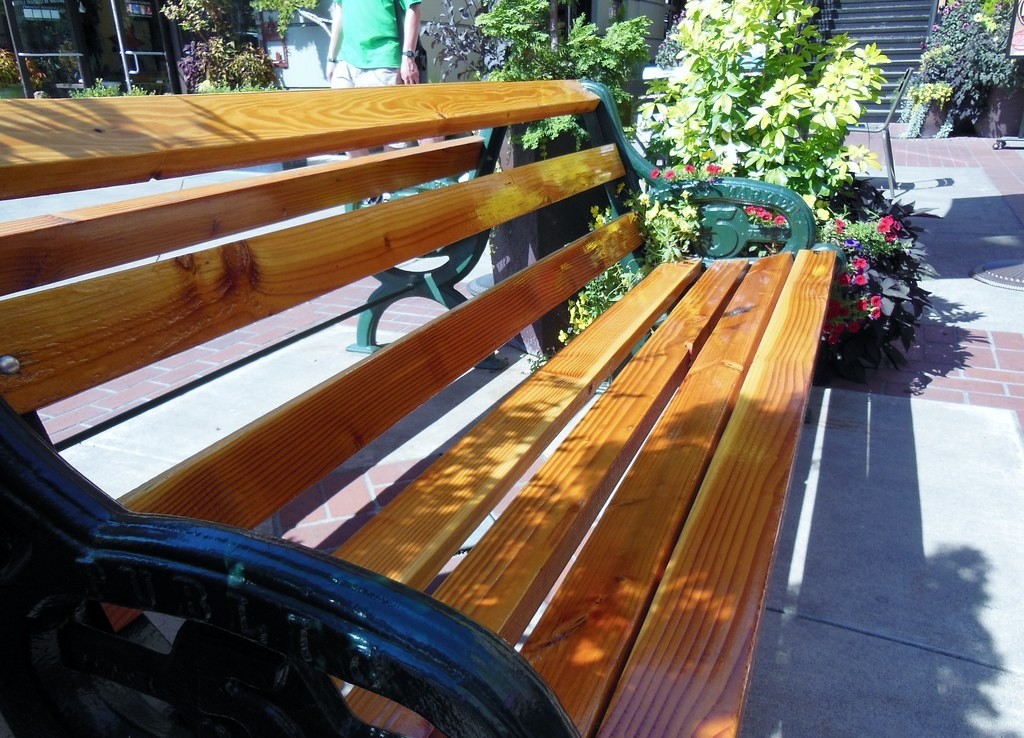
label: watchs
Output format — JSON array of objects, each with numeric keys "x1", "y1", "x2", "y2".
[{"x1": 402, "y1": 51, "x2": 416, "y2": 58}]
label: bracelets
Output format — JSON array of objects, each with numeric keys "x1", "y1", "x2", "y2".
[{"x1": 327, "y1": 58, "x2": 336, "y2": 62}]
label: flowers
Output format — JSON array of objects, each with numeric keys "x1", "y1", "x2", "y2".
[{"x1": 591, "y1": 163, "x2": 902, "y2": 347}]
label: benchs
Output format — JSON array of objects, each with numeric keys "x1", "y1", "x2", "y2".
[{"x1": 0, "y1": 81, "x2": 845, "y2": 738}]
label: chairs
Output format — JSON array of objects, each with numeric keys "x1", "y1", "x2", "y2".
[{"x1": 846, "y1": 68, "x2": 914, "y2": 198}]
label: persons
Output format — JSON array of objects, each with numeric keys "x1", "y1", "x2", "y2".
[{"x1": 326, "y1": 0, "x2": 423, "y2": 205}]
